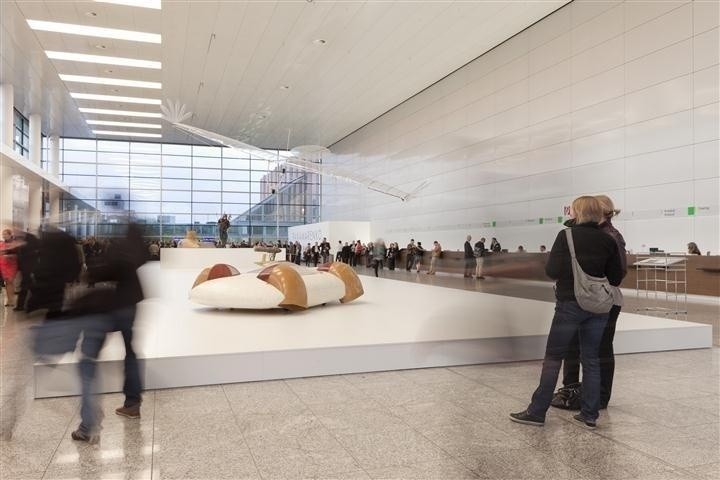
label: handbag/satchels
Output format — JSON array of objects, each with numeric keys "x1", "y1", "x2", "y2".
[
  {"x1": 551, "y1": 382, "x2": 582, "y2": 408},
  {"x1": 570, "y1": 261, "x2": 614, "y2": 314}
]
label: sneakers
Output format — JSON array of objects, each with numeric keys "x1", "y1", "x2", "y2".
[
  {"x1": 573, "y1": 414, "x2": 597, "y2": 430},
  {"x1": 509, "y1": 410, "x2": 545, "y2": 426},
  {"x1": 72, "y1": 431, "x2": 99, "y2": 443},
  {"x1": 116, "y1": 407, "x2": 140, "y2": 419}
]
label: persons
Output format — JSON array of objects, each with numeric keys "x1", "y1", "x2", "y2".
[
  {"x1": 688, "y1": 242, "x2": 701, "y2": 255},
  {"x1": 515, "y1": 245, "x2": 527, "y2": 253},
  {"x1": 335, "y1": 240, "x2": 362, "y2": 268},
  {"x1": 231, "y1": 240, "x2": 302, "y2": 264},
  {"x1": 509, "y1": 196, "x2": 621, "y2": 430},
  {"x1": 0, "y1": 215, "x2": 151, "y2": 445},
  {"x1": 149, "y1": 215, "x2": 230, "y2": 259},
  {"x1": 562, "y1": 196, "x2": 627, "y2": 409},
  {"x1": 304, "y1": 237, "x2": 330, "y2": 267},
  {"x1": 362, "y1": 239, "x2": 398, "y2": 277},
  {"x1": 463, "y1": 236, "x2": 500, "y2": 279},
  {"x1": 427, "y1": 241, "x2": 441, "y2": 275},
  {"x1": 406, "y1": 239, "x2": 424, "y2": 273},
  {"x1": 539, "y1": 245, "x2": 547, "y2": 253}
]
